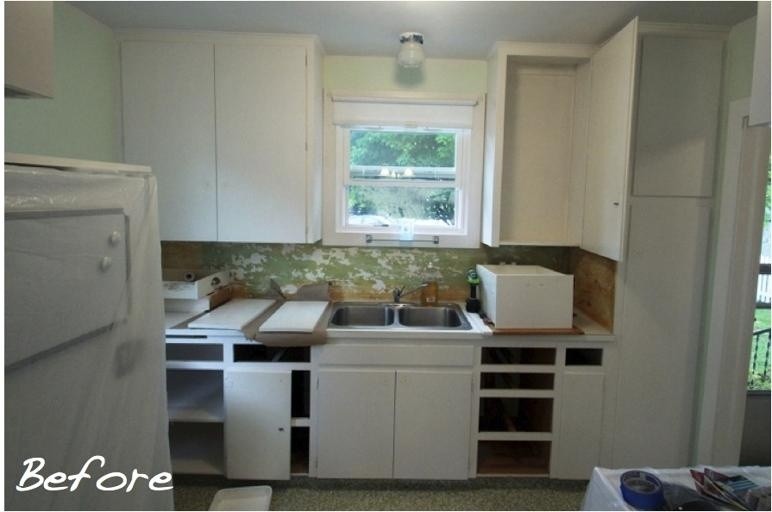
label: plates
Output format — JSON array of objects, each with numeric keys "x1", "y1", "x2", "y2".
[
  {"x1": 410, "y1": 167, "x2": 454, "y2": 178},
  {"x1": 350, "y1": 164, "x2": 381, "y2": 177}
]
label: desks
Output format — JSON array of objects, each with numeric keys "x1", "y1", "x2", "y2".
[{"x1": 579, "y1": 465, "x2": 770, "y2": 511}]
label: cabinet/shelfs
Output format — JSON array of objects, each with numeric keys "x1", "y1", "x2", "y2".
[
  {"x1": 314, "y1": 365, "x2": 475, "y2": 482},
  {"x1": 482, "y1": 14, "x2": 639, "y2": 264},
  {"x1": 164, "y1": 334, "x2": 313, "y2": 481},
  {"x1": 113, "y1": 27, "x2": 323, "y2": 245},
  {"x1": 478, "y1": 344, "x2": 612, "y2": 482},
  {"x1": 572, "y1": 32, "x2": 727, "y2": 470}
]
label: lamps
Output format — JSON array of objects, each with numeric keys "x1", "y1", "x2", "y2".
[{"x1": 397, "y1": 31, "x2": 426, "y2": 70}]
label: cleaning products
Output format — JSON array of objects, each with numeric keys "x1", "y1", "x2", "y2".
[{"x1": 465, "y1": 269, "x2": 481, "y2": 312}]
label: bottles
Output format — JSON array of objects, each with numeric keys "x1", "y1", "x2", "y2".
[{"x1": 420, "y1": 275, "x2": 438, "y2": 306}]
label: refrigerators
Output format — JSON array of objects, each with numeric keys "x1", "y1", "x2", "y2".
[{"x1": 5, "y1": 151, "x2": 176, "y2": 511}]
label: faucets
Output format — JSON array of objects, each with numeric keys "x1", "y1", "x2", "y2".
[{"x1": 392, "y1": 283, "x2": 430, "y2": 303}]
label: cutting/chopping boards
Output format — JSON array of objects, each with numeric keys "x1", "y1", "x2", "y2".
[
  {"x1": 257, "y1": 300, "x2": 329, "y2": 333},
  {"x1": 185, "y1": 298, "x2": 277, "y2": 331}
]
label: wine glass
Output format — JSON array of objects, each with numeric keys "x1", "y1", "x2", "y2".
[{"x1": 386, "y1": 166, "x2": 405, "y2": 179}]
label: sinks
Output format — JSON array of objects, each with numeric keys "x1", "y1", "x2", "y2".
[
  {"x1": 328, "y1": 301, "x2": 395, "y2": 329},
  {"x1": 398, "y1": 302, "x2": 471, "y2": 330}
]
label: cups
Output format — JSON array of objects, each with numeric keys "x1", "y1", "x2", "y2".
[{"x1": 398, "y1": 218, "x2": 415, "y2": 241}]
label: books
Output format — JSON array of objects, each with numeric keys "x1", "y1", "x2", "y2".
[{"x1": 690, "y1": 466, "x2": 771, "y2": 511}]
